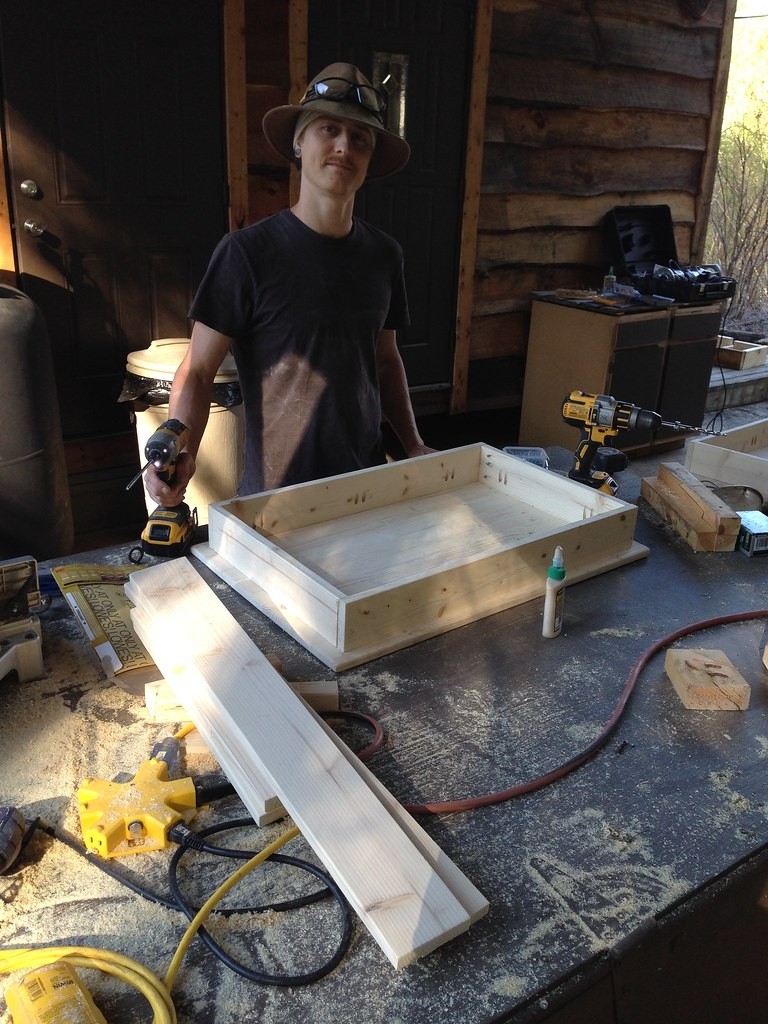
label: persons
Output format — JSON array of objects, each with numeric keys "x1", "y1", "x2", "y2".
[{"x1": 142, "y1": 62, "x2": 439, "y2": 508}]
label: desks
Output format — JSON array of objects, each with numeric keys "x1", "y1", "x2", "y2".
[{"x1": 0, "y1": 445, "x2": 768, "y2": 1024}]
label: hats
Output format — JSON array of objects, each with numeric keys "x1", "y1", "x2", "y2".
[{"x1": 262, "y1": 62, "x2": 411, "y2": 186}]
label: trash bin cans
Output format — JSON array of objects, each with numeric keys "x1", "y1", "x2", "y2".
[{"x1": 124, "y1": 335, "x2": 246, "y2": 529}]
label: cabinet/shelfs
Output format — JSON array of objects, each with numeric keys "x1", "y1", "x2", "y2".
[{"x1": 518, "y1": 291, "x2": 726, "y2": 459}]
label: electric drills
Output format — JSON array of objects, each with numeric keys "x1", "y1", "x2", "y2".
[
  {"x1": 126, "y1": 419, "x2": 199, "y2": 565},
  {"x1": 560, "y1": 389, "x2": 727, "y2": 496}
]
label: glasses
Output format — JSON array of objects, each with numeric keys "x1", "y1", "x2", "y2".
[{"x1": 306, "y1": 77, "x2": 387, "y2": 114}]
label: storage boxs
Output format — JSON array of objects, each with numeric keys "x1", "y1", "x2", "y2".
[
  {"x1": 684, "y1": 418, "x2": 768, "y2": 496},
  {"x1": 713, "y1": 335, "x2": 768, "y2": 370},
  {"x1": 604, "y1": 204, "x2": 738, "y2": 303},
  {"x1": 736, "y1": 511, "x2": 768, "y2": 558},
  {"x1": 190, "y1": 441, "x2": 651, "y2": 673}
]
